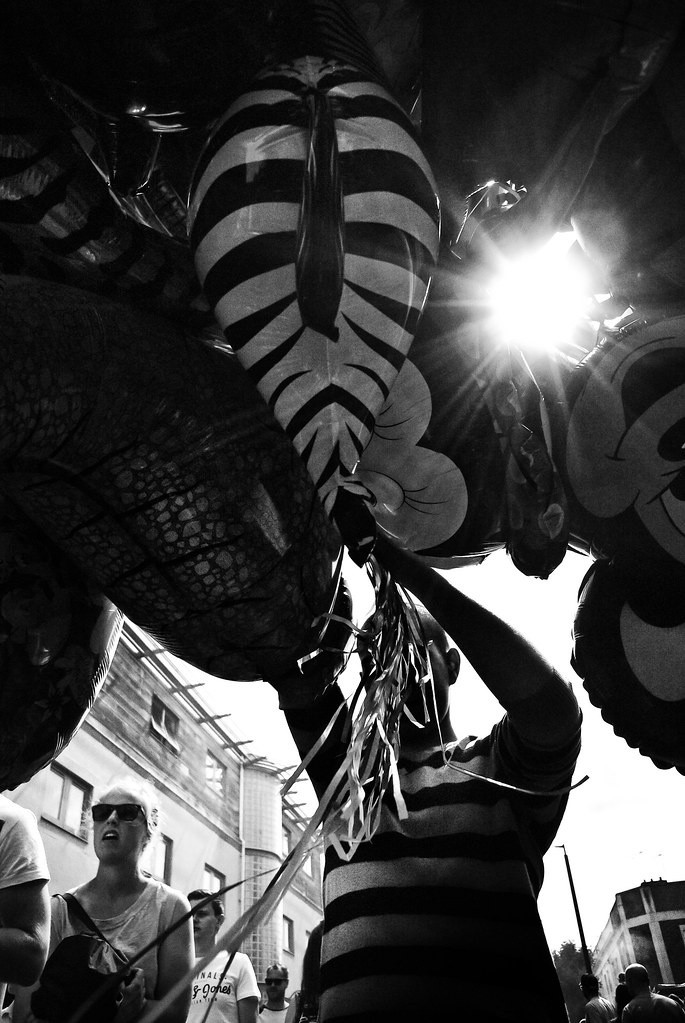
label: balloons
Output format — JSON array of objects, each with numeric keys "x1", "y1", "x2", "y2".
[{"x1": 0, "y1": 1, "x2": 684, "y2": 798}]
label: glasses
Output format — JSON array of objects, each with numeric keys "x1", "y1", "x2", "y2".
[
  {"x1": 91, "y1": 804, "x2": 145, "y2": 822},
  {"x1": 265, "y1": 978, "x2": 288, "y2": 986}
]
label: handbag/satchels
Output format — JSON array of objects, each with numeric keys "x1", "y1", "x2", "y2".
[{"x1": 30, "y1": 934, "x2": 135, "y2": 1023}]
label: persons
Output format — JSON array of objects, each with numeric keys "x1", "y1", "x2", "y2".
[
  {"x1": 261, "y1": 500, "x2": 582, "y2": 1022},
  {"x1": 567, "y1": 962, "x2": 685, "y2": 1023},
  {"x1": 185, "y1": 886, "x2": 261, "y2": 1023},
  {"x1": 258, "y1": 963, "x2": 291, "y2": 1023},
  {"x1": 0, "y1": 798, "x2": 51, "y2": 1023},
  {"x1": 51, "y1": 788, "x2": 197, "y2": 1023}
]
